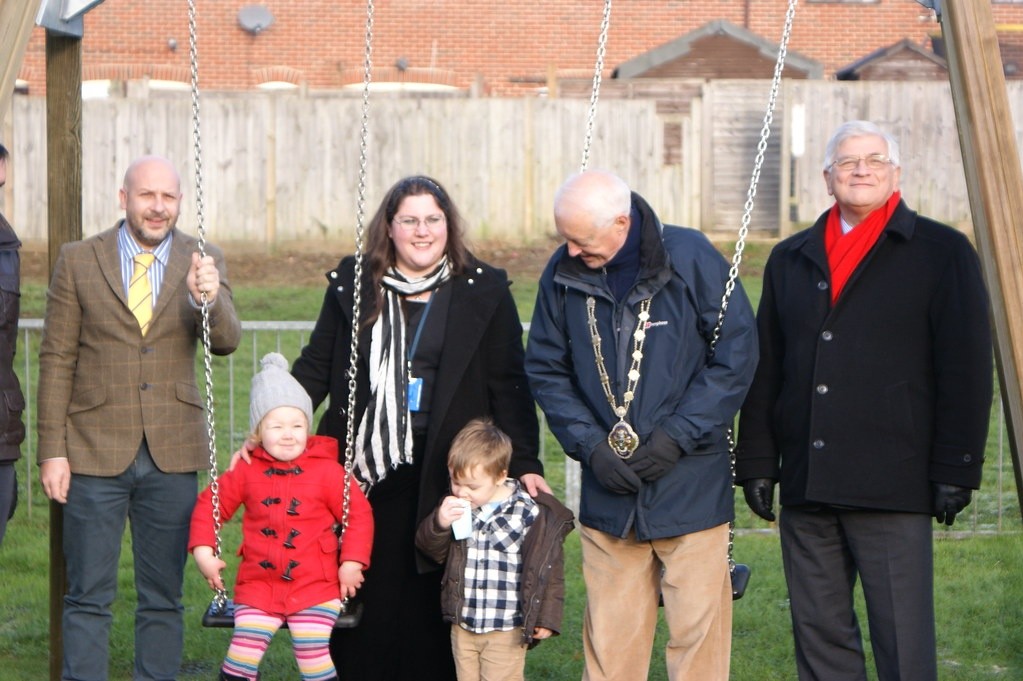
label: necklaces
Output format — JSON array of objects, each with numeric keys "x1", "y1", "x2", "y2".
[
  {"x1": 415, "y1": 293, "x2": 422, "y2": 300},
  {"x1": 585, "y1": 292, "x2": 653, "y2": 459}
]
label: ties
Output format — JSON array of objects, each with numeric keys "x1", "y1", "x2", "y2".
[{"x1": 128, "y1": 253, "x2": 155, "y2": 337}]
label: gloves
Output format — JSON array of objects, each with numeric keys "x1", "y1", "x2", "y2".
[
  {"x1": 742, "y1": 478, "x2": 775, "y2": 521},
  {"x1": 932, "y1": 482, "x2": 972, "y2": 525},
  {"x1": 589, "y1": 441, "x2": 643, "y2": 494},
  {"x1": 625, "y1": 431, "x2": 682, "y2": 483}
]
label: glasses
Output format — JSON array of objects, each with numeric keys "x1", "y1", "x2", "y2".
[
  {"x1": 828, "y1": 153, "x2": 896, "y2": 170},
  {"x1": 392, "y1": 214, "x2": 447, "y2": 231}
]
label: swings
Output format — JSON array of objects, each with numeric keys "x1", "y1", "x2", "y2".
[
  {"x1": 579, "y1": 0, "x2": 798, "y2": 607},
  {"x1": 188, "y1": 0, "x2": 381, "y2": 629}
]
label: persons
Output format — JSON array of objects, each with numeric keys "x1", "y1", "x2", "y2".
[
  {"x1": 413, "y1": 419, "x2": 575, "y2": 681},
  {"x1": 734, "y1": 121, "x2": 993, "y2": 681},
  {"x1": 225, "y1": 176, "x2": 555, "y2": 681},
  {"x1": 36, "y1": 155, "x2": 241, "y2": 681},
  {"x1": 523, "y1": 170, "x2": 760, "y2": 681},
  {"x1": 0, "y1": 144, "x2": 25, "y2": 542},
  {"x1": 188, "y1": 351, "x2": 373, "y2": 681}
]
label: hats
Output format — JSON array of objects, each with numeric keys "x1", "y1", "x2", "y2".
[{"x1": 249, "y1": 352, "x2": 312, "y2": 435}]
